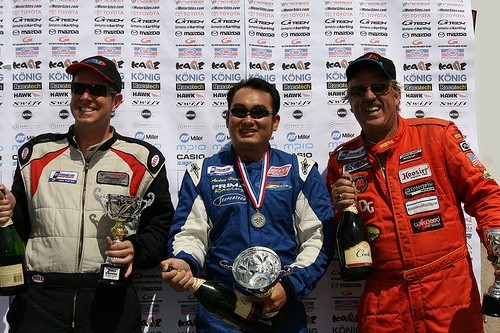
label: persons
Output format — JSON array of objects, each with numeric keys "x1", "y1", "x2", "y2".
[
  {"x1": 160, "y1": 77, "x2": 335, "y2": 333},
  {"x1": 0, "y1": 55, "x2": 175, "y2": 333},
  {"x1": 326, "y1": 52, "x2": 500, "y2": 333}
]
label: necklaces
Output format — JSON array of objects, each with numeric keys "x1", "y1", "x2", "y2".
[{"x1": 365, "y1": 138, "x2": 376, "y2": 144}]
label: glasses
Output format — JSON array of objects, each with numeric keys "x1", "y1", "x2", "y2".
[
  {"x1": 70, "y1": 82, "x2": 116, "y2": 97},
  {"x1": 229, "y1": 108, "x2": 273, "y2": 119},
  {"x1": 349, "y1": 84, "x2": 391, "y2": 98}
]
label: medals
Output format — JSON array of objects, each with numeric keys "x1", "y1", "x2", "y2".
[{"x1": 251, "y1": 213, "x2": 266, "y2": 228}]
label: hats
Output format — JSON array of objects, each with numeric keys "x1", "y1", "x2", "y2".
[
  {"x1": 66, "y1": 56, "x2": 122, "y2": 93},
  {"x1": 346, "y1": 52, "x2": 396, "y2": 84}
]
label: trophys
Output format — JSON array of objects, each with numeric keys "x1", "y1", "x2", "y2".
[
  {"x1": 220, "y1": 245, "x2": 293, "y2": 318},
  {"x1": 93, "y1": 187, "x2": 155, "y2": 284}
]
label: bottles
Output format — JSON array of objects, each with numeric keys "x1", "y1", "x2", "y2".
[
  {"x1": 337, "y1": 177, "x2": 373, "y2": 281},
  {"x1": 0, "y1": 190, "x2": 29, "y2": 296},
  {"x1": 166, "y1": 265, "x2": 263, "y2": 330}
]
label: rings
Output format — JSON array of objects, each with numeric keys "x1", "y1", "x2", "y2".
[{"x1": 336, "y1": 193, "x2": 342, "y2": 201}]
